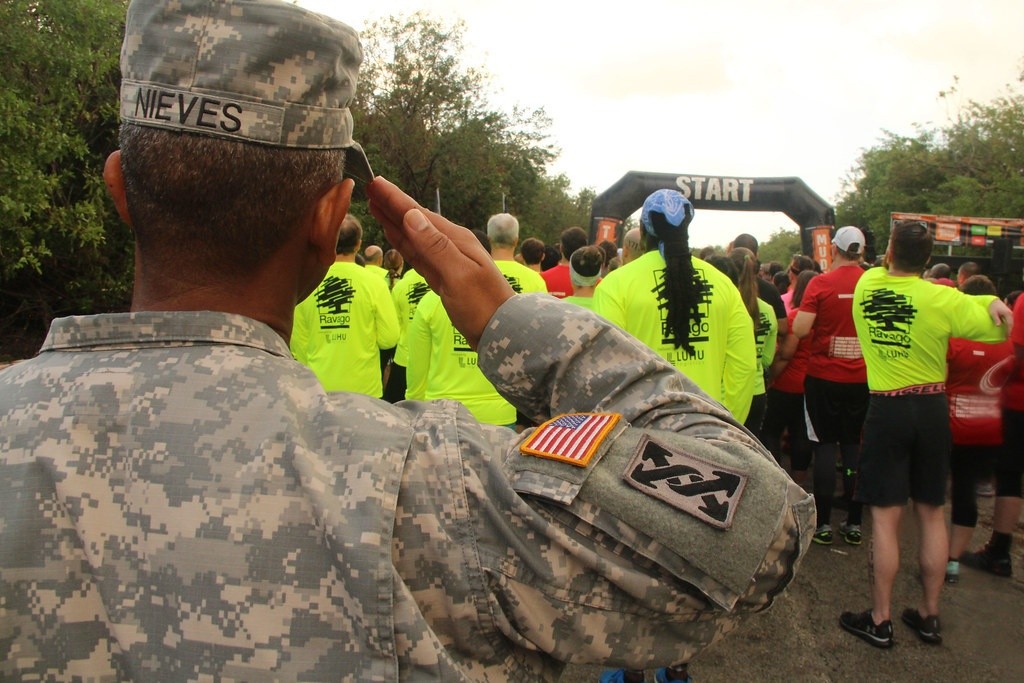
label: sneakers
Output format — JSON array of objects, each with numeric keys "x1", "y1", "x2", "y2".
[
  {"x1": 599, "y1": 668, "x2": 644, "y2": 683},
  {"x1": 901, "y1": 606, "x2": 941, "y2": 643},
  {"x1": 841, "y1": 610, "x2": 893, "y2": 648},
  {"x1": 654, "y1": 665, "x2": 690, "y2": 683}
]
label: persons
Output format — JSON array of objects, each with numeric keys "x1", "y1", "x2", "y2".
[
  {"x1": 593, "y1": 188, "x2": 757, "y2": 683},
  {"x1": 701, "y1": 234, "x2": 824, "y2": 487},
  {"x1": 516, "y1": 227, "x2": 642, "y2": 308},
  {"x1": 862, "y1": 229, "x2": 885, "y2": 267},
  {"x1": 405, "y1": 230, "x2": 517, "y2": 432},
  {"x1": 289, "y1": 213, "x2": 400, "y2": 399},
  {"x1": 792, "y1": 225, "x2": 866, "y2": 544},
  {"x1": 959, "y1": 290, "x2": 1024, "y2": 577},
  {"x1": 839, "y1": 219, "x2": 1014, "y2": 648},
  {"x1": 355, "y1": 245, "x2": 431, "y2": 403},
  {"x1": 923, "y1": 262, "x2": 981, "y2": 286},
  {"x1": 913, "y1": 276, "x2": 1017, "y2": 584},
  {"x1": 0, "y1": 0, "x2": 816, "y2": 683},
  {"x1": 486, "y1": 213, "x2": 547, "y2": 297}
]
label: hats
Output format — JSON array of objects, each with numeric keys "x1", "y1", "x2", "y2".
[
  {"x1": 118, "y1": 1, "x2": 376, "y2": 184},
  {"x1": 829, "y1": 228, "x2": 865, "y2": 256}
]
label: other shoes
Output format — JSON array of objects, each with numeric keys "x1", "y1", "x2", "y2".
[
  {"x1": 979, "y1": 543, "x2": 1013, "y2": 576},
  {"x1": 946, "y1": 561, "x2": 960, "y2": 584},
  {"x1": 812, "y1": 528, "x2": 832, "y2": 544},
  {"x1": 837, "y1": 523, "x2": 862, "y2": 545}
]
